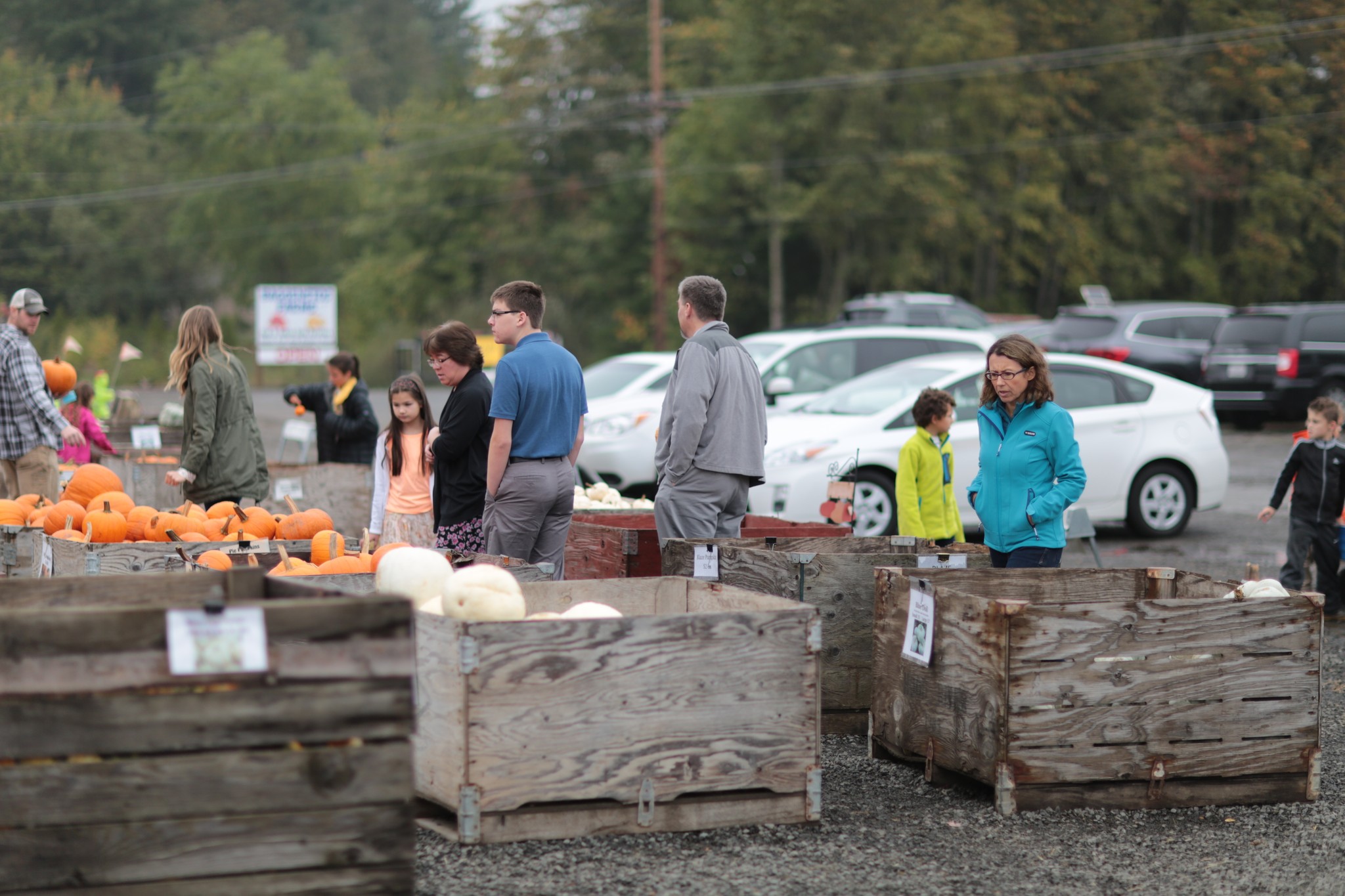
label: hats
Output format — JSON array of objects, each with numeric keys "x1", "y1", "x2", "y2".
[{"x1": 9, "y1": 288, "x2": 50, "y2": 316}]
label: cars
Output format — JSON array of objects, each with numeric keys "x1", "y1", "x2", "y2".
[{"x1": 746, "y1": 351, "x2": 1233, "y2": 552}]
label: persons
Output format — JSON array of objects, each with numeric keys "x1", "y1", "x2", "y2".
[
  {"x1": 653, "y1": 273, "x2": 769, "y2": 554},
  {"x1": 481, "y1": 281, "x2": 587, "y2": 581},
  {"x1": 0, "y1": 287, "x2": 86, "y2": 504},
  {"x1": 282, "y1": 353, "x2": 378, "y2": 469},
  {"x1": 423, "y1": 319, "x2": 494, "y2": 555},
  {"x1": 967, "y1": 334, "x2": 1087, "y2": 569},
  {"x1": 360, "y1": 373, "x2": 438, "y2": 551},
  {"x1": 59, "y1": 384, "x2": 116, "y2": 466},
  {"x1": 1257, "y1": 397, "x2": 1345, "y2": 621},
  {"x1": 164, "y1": 303, "x2": 271, "y2": 516},
  {"x1": 1292, "y1": 387, "x2": 1345, "y2": 593},
  {"x1": 895, "y1": 387, "x2": 966, "y2": 548}
]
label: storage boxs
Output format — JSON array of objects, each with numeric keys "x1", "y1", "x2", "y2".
[
  {"x1": 407, "y1": 501, "x2": 1328, "y2": 842},
  {"x1": 1, "y1": 534, "x2": 418, "y2": 895}
]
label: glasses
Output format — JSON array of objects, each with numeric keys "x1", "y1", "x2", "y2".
[
  {"x1": 427, "y1": 356, "x2": 453, "y2": 367},
  {"x1": 984, "y1": 368, "x2": 1028, "y2": 380},
  {"x1": 491, "y1": 311, "x2": 528, "y2": 317}
]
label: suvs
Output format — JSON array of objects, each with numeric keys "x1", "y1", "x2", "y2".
[
  {"x1": 1197, "y1": 303, "x2": 1345, "y2": 425},
  {"x1": 573, "y1": 326, "x2": 1002, "y2": 499},
  {"x1": 1045, "y1": 303, "x2": 1236, "y2": 387},
  {"x1": 843, "y1": 293, "x2": 992, "y2": 334}
]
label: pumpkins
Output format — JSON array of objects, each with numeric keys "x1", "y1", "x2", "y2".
[
  {"x1": 38, "y1": 356, "x2": 77, "y2": 401},
  {"x1": 1, "y1": 470, "x2": 410, "y2": 666}
]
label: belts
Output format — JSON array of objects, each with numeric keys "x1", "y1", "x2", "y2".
[{"x1": 510, "y1": 456, "x2": 558, "y2": 464}]
